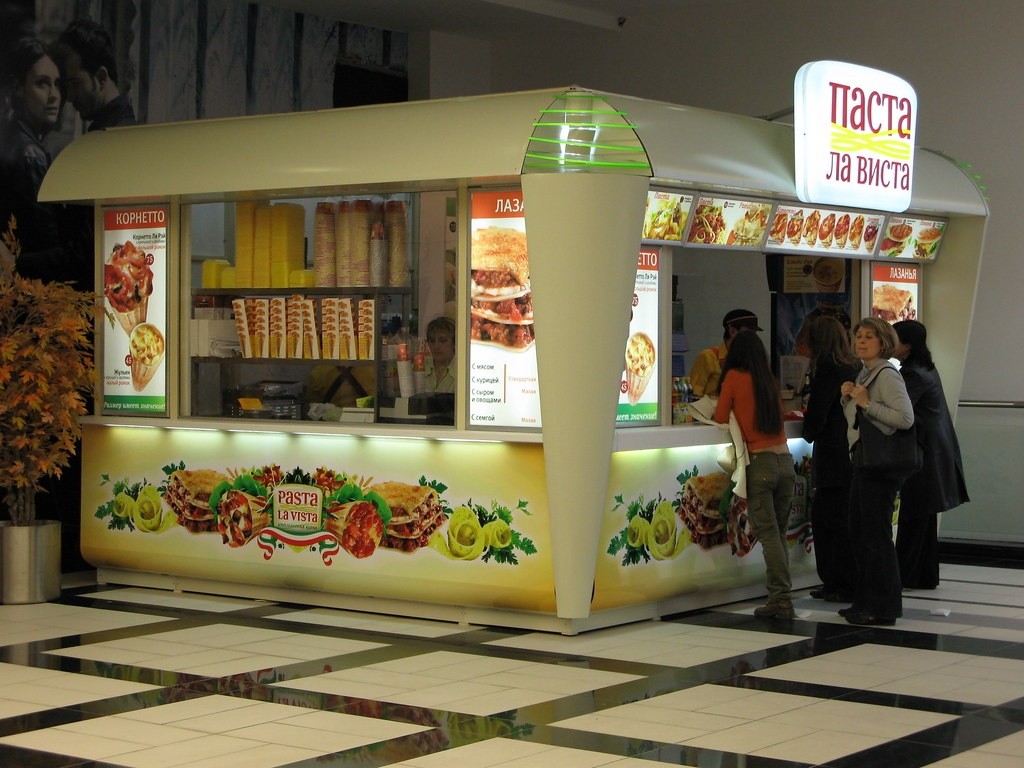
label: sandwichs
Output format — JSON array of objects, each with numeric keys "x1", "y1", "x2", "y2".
[
  {"x1": 871, "y1": 285, "x2": 917, "y2": 325},
  {"x1": 165, "y1": 470, "x2": 237, "y2": 535},
  {"x1": 678, "y1": 472, "x2": 731, "y2": 550},
  {"x1": 360, "y1": 480, "x2": 447, "y2": 553}
]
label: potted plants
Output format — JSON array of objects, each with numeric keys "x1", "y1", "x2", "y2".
[{"x1": 0, "y1": 214, "x2": 120, "y2": 606}]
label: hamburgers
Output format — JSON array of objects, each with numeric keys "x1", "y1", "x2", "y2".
[
  {"x1": 880, "y1": 224, "x2": 942, "y2": 259},
  {"x1": 467, "y1": 225, "x2": 535, "y2": 353}
]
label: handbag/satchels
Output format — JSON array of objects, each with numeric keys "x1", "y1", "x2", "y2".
[{"x1": 853, "y1": 366, "x2": 918, "y2": 470}]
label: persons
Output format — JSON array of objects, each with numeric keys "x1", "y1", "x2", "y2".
[
  {"x1": 715, "y1": 331, "x2": 796, "y2": 621},
  {"x1": 689, "y1": 310, "x2": 764, "y2": 396},
  {"x1": 838, "y1": 317, "x2": 915, "y2": 625},
  {"x1": 891, "y1": 320, "x2": 970, "y2": 590},
  {"x1": 427, "y1": 316, "x2": 456, "y2": 391},
  {"x1": 0, "y1": 17, "x2": 135, "y2": 248},
  {"x1": 306, "y1": 364, "x2": 377, "y2": 406},
  {"x1": 802, "y1": 315, "x2": 862, "y2": 601}
]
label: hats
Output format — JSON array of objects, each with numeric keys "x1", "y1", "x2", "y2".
[{"x1": 724, "y1": 309, "x2": 764, "y2": 331}]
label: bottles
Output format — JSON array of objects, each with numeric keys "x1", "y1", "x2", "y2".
[{"x1": 672, "y1": 376, "x2": 694, "y2": 425}]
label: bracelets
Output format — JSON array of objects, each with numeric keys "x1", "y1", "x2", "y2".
[{"x1": 863, "y1": 402, "x2": 870, "y2": 409}]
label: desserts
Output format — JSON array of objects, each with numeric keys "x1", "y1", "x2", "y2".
[
  {"x1": 623, "y1": 331, "x2": 656, "y2": 407},
  {"x1": 769, "y1": 210, "x2": 880, "y2": 254},
  {"x1": 212, "y1": 465, "x2": 384, "y2": 558},
  {"x1": 104, "y1": 240, "x2": 154, "y2": 338}
]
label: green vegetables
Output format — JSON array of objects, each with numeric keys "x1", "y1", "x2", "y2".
[
  {"x1": 93, "y1": 459, "x2": 188, "y2": 532},
  {"x1": 417, "y1": 477, "x2": 538, "y2": 566},
  {"x1": 604, "y1": 465, "x2": 700, "y2": 566}
]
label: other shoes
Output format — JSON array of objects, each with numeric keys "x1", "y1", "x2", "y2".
[
  {"x1": 810, "y1": 587, "x2": 857, "y2": 603},
  {"x1": 754, "y1": 594, "x2": 794, "y2": 620},
  {"x1": 838, "y1": 604, "x2": 865, "y2": 616},
  {"x1": 846, "y1": 609, "x2": 896, "y2": 626}
]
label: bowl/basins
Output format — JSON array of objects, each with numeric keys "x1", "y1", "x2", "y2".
[
  {"x1": 314, "y1": 199, "x2": 409, "y2": 288},
  {"x1": 812, "y1": 257, "x2": 844, "y2": 293}
]
